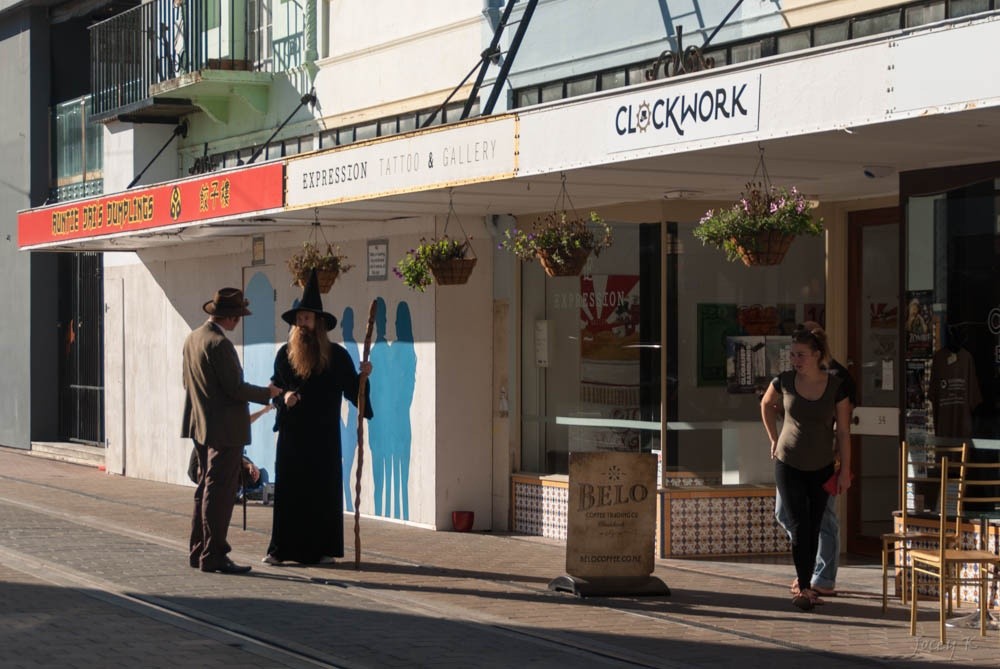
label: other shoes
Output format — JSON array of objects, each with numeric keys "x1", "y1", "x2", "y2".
[
  {"x1": 262, "y1": 553, "x2": 285, "y2": 565},
  {"x1": 319, "y1": 556, "x2": 336, "y2": 563}
]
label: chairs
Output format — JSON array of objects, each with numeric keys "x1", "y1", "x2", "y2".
[
  {"x1": 877, "y1": 438, "x2": 969, "y2": 619},
  {"x1": 907, "y1": 456, "x2": 1000, "y2": 646}
]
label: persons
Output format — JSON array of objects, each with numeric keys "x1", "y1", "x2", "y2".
[
  {"x1": 179, "y1": 287, "x2": 283, "y2": 574},
  {"x1": 187, "y1": 443, "x2": 260, "y2": 501},
  {"x1": 799, "y1": 319, "x2": 849, "y2": 597},
  {"x1": 760, "y1": 326, "x2": 848, "y2": 610},
  {"x1": 261, "y1": 274, "x2": 373, "y2": 566}
]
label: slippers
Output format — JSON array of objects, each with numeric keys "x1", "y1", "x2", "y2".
[
  {"x1": 791, "y1": 584, "x2": 800, "y2": 593},
  {"x1": 806, "y1": 591, "x2": 825, "y2": 604},
  {"x1": 792, "y1": 592, "x2": 815, "y2": 611},
  {"x1": 811, "y1": 587, "x2": 837, "y2": 597}
]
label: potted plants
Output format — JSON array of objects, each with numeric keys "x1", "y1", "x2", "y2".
[
  {"x1": 495, "y1": 206, "x2": 614, "y2": 278},
  {"x1": 396, "y1": 235, "x2": 479, "y2": 292},
  {"x1": 283, "y1": 239, "x2": 354, "y2": 295},
  {"x1": 693, "y1": 183, "x2": 826, "y2": 267}
]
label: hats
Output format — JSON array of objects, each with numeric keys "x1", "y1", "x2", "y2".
[
  {"x1": 280, "y1": 270, "x2": 338, "y2": 333},
  {"x1": 202, "y1": 288, "x2": 254, "y2": 318}
]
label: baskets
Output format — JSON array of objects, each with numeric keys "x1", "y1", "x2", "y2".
[
  {"x1": 427, "y1": 255, "x2": 478, "y2": 286},
  {"x1": 292, "y1": 267, "x2": 342, "y2": 294},
  {"x1": 726, "y1": 230, "x2": 798, "y2": 266},
  {"x1": 533, "y1": 237, "x2": 590, "y2": 277}
]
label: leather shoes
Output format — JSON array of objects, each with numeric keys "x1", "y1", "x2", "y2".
[{"x1": 201, "y1": 557, "x2": 252, "y2": 574}]
